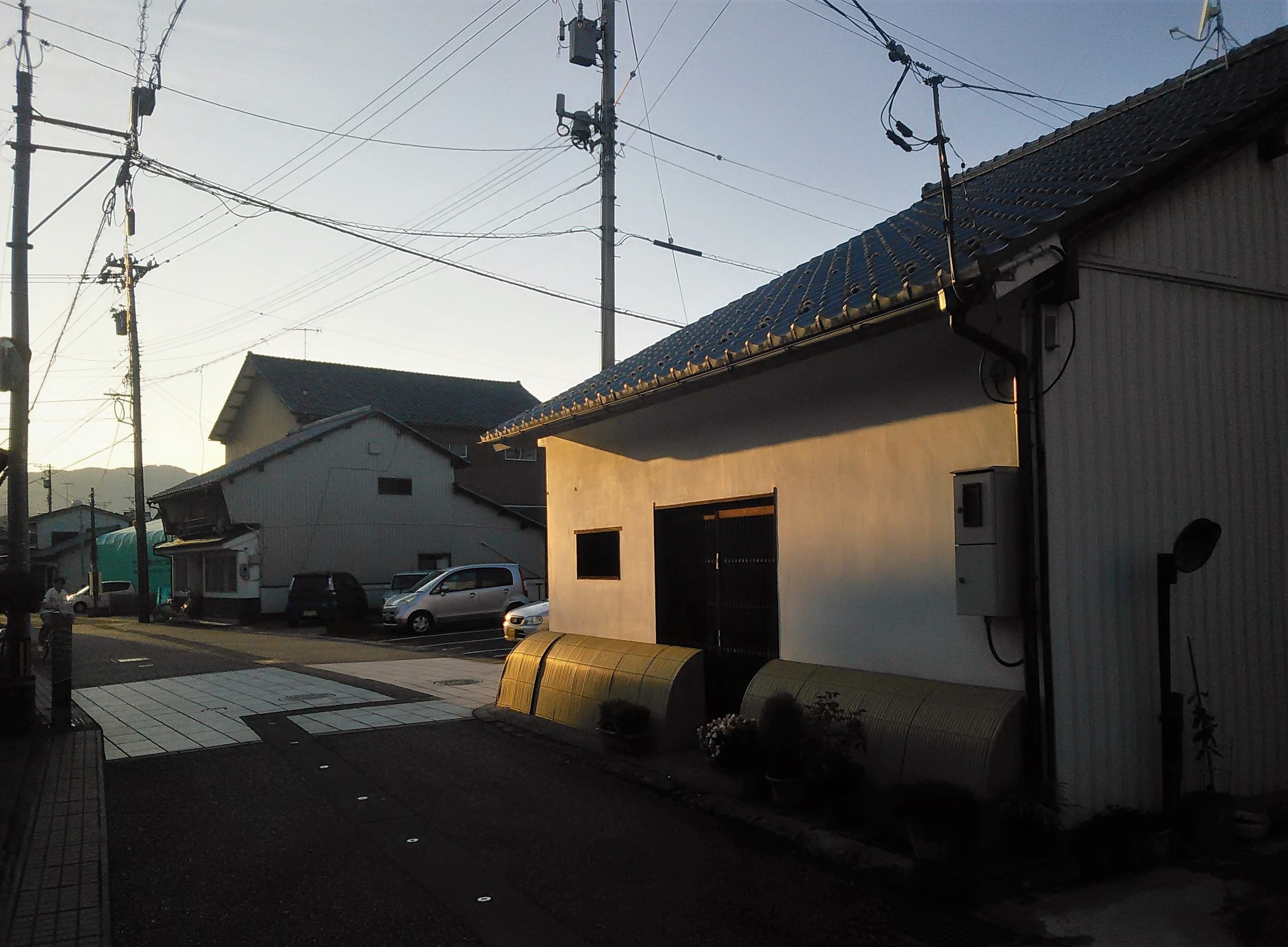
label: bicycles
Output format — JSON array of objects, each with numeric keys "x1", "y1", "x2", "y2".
[{"x1": 39, "y1": 608, "x2": 61, "y2": 659}]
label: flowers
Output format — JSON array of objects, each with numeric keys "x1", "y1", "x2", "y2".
[{"x1": 696, "y1": 713, "x2": 760, "y2": 774}]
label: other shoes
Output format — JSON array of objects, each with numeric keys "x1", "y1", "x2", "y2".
[{"x1": 38, "y1": 645, "x2": 43, "y2": 652}]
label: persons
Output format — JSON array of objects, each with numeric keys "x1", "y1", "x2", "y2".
[{"x1": 37, "y1": 577, "x2": 68, "y2": 653}]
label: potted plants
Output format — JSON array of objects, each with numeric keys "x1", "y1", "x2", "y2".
[
  {"x1": 596, "y1": 697, "x2": 652, "y2": 756},
  {"x1": 1179, "y1": 634, "x2": 1237, "y2": 852},
  {"x1": 757, "y1": 688, "x2": 982, "y2": 866},
  {"x1": 1234, "y1": 809, "x2": 1268, "y2": 842},
  {"x1": 1092, "y1": 803, "x2": 1171, "y2": 868}
]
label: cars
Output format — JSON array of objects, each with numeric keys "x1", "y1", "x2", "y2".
[
  {"x1": 284, "y1": 570, "x2": 369, "y2": 629},
  {"x1": 71, "y1": 580, "x2": 136, "y2": 614},
  {"x1": 379, "y1": 562, "x2": 531, "y2": 637},
  {"x1": 502, "y1": 597, "x2": 549, "y2": 643}
]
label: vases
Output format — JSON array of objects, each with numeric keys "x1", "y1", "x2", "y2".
[{"x1": 730, "y1": 772, "x2": 767, "y2": 806}]
